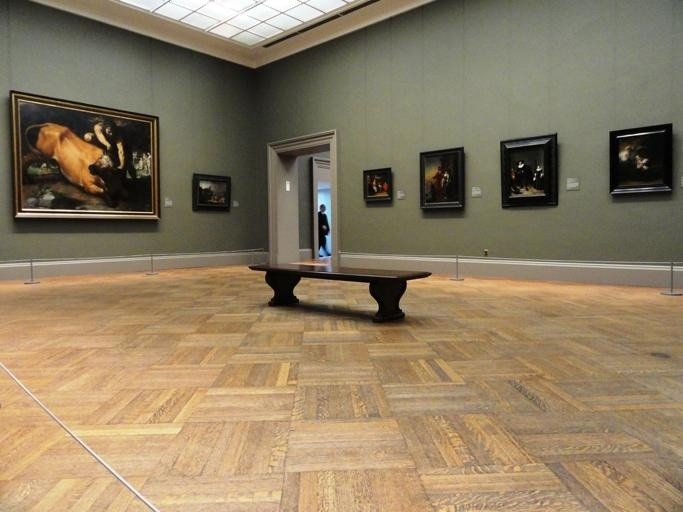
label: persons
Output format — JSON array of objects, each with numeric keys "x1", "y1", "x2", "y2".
[
  {"x1": 83, "y1": 119, "x2": 125, "y2": 169},
  {"x1": 370, "y1": 145, "x2": 652, "y2": 199},
  {"x1": 318, "y1": 204, "x2": 331, "y2": 256}
]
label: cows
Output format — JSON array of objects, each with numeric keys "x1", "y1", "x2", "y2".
[{"x1": 22, "y1": 122, "x2": 117, "y2": 208}]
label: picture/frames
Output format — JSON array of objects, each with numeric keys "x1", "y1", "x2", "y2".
[
  {"x1": 419, "y1": 146, "x2": 466, "y2": 211},
  {"x1": 362, "y1": 167, "x2": 393, "y2": 203},
  {"x1": 499, "y1": 132, "x2": 559, "y2": 209},
  {"x1": 8, "y1": 89, "x2": 162, "y2": 221},
  {"x1": 608, "y1": 121, "x2": 675, "y2": 198},
  {"x1": 192, "y1": 172, "x2": 231, "y2": 211}
]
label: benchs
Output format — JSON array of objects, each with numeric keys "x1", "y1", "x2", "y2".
[{"x1": 246, "y1": 262, "x2": 432, "y2": 324}]
